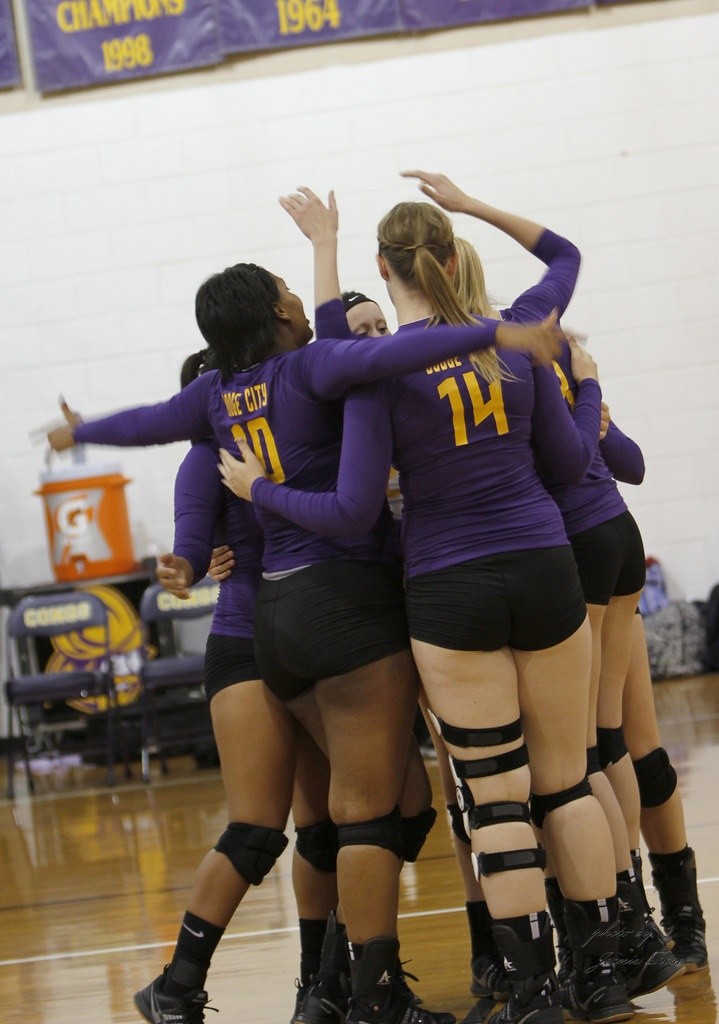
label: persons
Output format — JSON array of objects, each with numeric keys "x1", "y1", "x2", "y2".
[
  {"x1": 51, "y1": 265, "x2": 568, "y2": 1024},
  {"x1": 133, "y1": 172, "x2": 708, "y2": 1024}
]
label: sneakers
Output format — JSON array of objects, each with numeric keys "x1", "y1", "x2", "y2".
[{"x1": 133, "y1": 850, "x2": 710, "y2": 1024}]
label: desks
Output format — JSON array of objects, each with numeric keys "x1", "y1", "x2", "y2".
[{"x1": 0, "y1": 566, "x2": 179, "y2": 754}]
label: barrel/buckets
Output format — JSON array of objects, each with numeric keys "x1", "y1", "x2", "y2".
[{"x1": 32, "y1": 442, "x2": 137, "y2": 581}]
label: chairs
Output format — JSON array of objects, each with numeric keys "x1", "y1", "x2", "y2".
[
  {"x1": 4, "y1": 593, "x2": 119, "y2": 801},
  {"x1": 137, "y1": 574, "x2": 222, "y2": 780}
]
